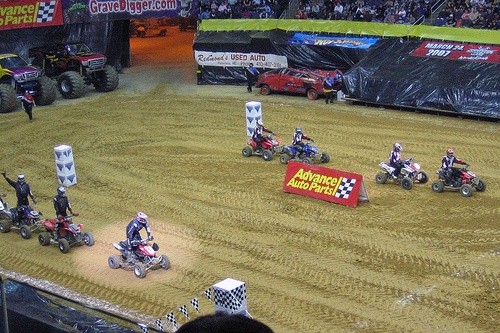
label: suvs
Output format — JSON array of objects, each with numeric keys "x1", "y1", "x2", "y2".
[
  {"x1": 0, "y1": 53, "x2": 56, "y2": 113},
  {"x1": 29, "y1": 42, "x2": 119, "y2": 99}
]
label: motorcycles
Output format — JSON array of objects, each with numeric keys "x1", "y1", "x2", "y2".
[
  {"x1": 1, "y1": 206, "x2": 45, "y2": 240},
  {"x1": 280, "y1": 139, "x2": 329, "y2": 166},
  {"x1": 108, "y1": 237, "x2": 171, "y2": 277},
  {"x1": 242, "y1": 132, "x2": 283, "y2": 160},
  {"x1": 431, "y1": 164, "x2": 487, "y2": 197},
  {"x1": 38, "y1": 213, "x2": 95, "y2": 255},
  {"x1": 375, "y1": 159, "x2": 429, "y2": 190}
]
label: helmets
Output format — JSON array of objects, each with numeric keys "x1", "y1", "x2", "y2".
[
  {"x1": 295, "y1": 128, "x2": 303, "y2": 133},
  {"x1": 136, "y1": 212, "x2": 148, "y2": 224},
  {"x1": 256, "y1": 120, "x2": 264, "y2": 127},
  {"x1": 17, "y1": 174, "x2": 25, "y2": 182},
  {"x1": 57, "y1": 186, "x2": 65, "y2": 198},
  {"x1": 446, "y1": 148, "x2": 454, "y2": 154},
  {"x1": 394, "y1": 143, "x2": 401, "y2": 150}
]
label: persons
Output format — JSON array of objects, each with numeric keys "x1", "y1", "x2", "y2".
[
  {"x1": 1, "y1": 171, "x2": 39, "y2": 209},
  {"x1": 21, "y1": 90, "x2": 36, "y2": 120},
  {"x1": 126, "y1": 212, "x2": 154, "y2": 250},
  {"x1": 176, "y1": 0, "x2": 500, "y2": 36},
  {"x1": 323, "y1": 72, "x2": 334, "y2": 103},
  {"x1": 442, "y1": 147, "x2": 470, "y2": 185},
  {"x1": 253, "y1": 119, "x2": 274, "y2": 149},
  {"x1": 246, "y1": 62, "x2": 260, "y2": 93},
  {"x1": 292, "y1": 126, "x2": 314, "y2": 154},
  {"x1": 53, "y1": 186, "x2": 79, "y2": 219},
  {"x1": 390, "y1": 143, "x2": 409, "y2": 179}
]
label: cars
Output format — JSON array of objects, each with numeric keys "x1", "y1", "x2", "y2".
[{"x1": 256, "y1": 68, "x2": 324, "y2": 100}]
